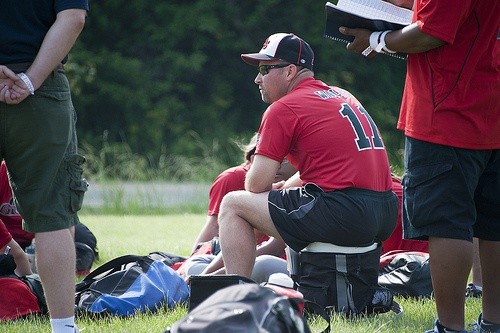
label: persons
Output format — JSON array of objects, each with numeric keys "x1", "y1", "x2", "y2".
[
  {"x1": 341, "y1": 0, "x2": 500, "y2": 333},
  {"x1": 0, "y1": 159, "x2": 98, "y2": 323},
  {"x1": 176, "y1": 32, "x2": 483, "y2": 323},
  {"x1": 0, "y1": 0, "x2": 91, "y2": 333}
]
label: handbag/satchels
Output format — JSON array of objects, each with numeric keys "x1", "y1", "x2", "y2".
[
  {"x1": 164, "y1": 282, "x2": 332, "y2": 333},
  {"x1": 74, "y1": 253, "x2": 189, "y2": 320},
  {"x1": 149, "y1": 251, "x2": 187, "y2": 271},
  {"x1": 376, "y1": 249, "x2": 435, "y2": 300},
  {"x1": 0, "y1": 272, "x2": 49, "y2": 324}
]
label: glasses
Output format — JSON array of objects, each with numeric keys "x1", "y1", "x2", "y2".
[{"x1": 257, "y1": 63, "x2": 290, "y2": 76}]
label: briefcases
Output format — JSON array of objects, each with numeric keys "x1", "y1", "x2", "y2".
[{"x1": 286, "y1": 241, "x2": 403, "y2": 316}]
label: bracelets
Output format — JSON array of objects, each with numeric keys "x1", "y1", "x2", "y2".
[
  {"x1": 362, "y1": 30, "x2": 397, "y2": 56},
  {"x1": 17, "y1": 73, "x2": 35, "y2": 96}
]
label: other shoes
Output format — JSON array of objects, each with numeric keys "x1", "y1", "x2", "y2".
[
  {"x1": 465, "y1": 283, "x2": 482, "y2": 300},
  {"x1": 469, "y1": 313, "x2": 500, "y2": 333},
  {"x1": 424, "y1": 319, "x2": 467, "y2": 333}
]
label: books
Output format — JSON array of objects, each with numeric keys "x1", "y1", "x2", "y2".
[{"x1": 325, "y1": 0, "x2": 414, "y2": 60}]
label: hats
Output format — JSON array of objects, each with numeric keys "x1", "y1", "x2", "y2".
[{"x1": 240, "y1": 32, "x2": 314, "y2": 71}]
